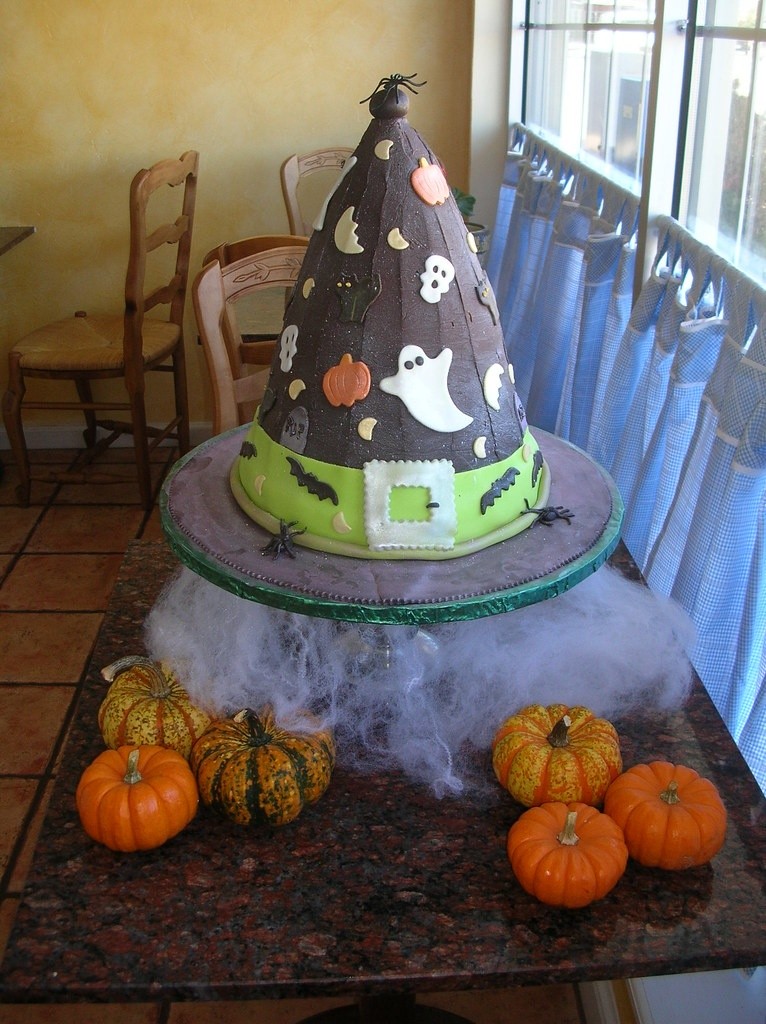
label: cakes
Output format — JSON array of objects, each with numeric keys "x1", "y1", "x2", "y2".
[{"x1": 225, "y1": 73, "x2": 551, "y2": 560}]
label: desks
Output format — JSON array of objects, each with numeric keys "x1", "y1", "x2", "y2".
[{"x1": 0, "y1": 533, "x2": 766, "y2": 1024}]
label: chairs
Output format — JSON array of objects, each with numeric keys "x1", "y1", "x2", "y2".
[
  {"x1": 193, "y1": 246, "x2": 309, "y2": 434},
  {"x1": 280, "y1": 147, "x2": 354, "y2": 235},
  {"x1": 202, "y1": 235, "x2": 309, "y2": 426},
  {"x1": 0, "y1": 150, "x2": 199, "y2": 510}
]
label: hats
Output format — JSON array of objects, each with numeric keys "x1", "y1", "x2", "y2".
[{"x1": 157, "y1": 73, "x2": 628, "y2": 627}]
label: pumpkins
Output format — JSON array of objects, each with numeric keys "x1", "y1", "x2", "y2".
[
  {"x1": 493, "y1": 705, "x2": 727, "y2": 906},
  {"x1": 77, "y1": 652, "x2": 335, "y2": 852}
]
